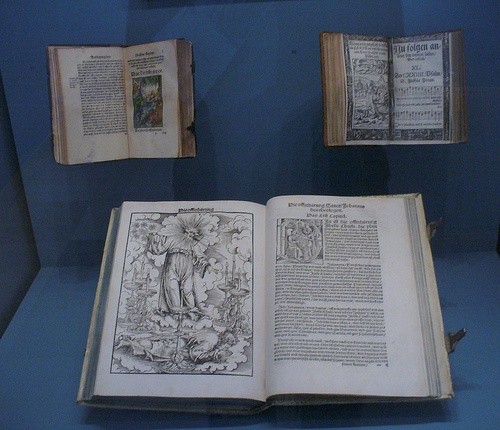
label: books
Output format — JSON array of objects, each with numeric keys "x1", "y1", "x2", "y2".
[
  {"x1": 76, "y1": 194, "x2": 454, "y2": 418},
  {"x1": 319, "y1": 28, "x2": 470, "y2": 149},
  {"x1": 48, "y1": 37, "x2": 196, "y2": 166}
]
